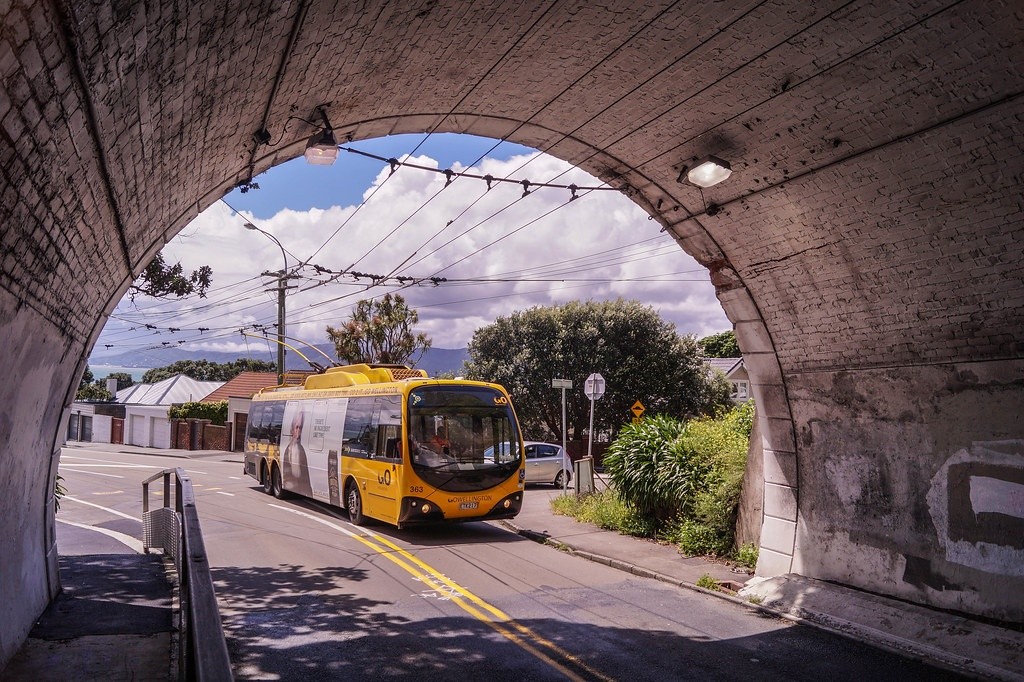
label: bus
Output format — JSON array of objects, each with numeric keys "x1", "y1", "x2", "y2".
[{"x1": 243, "y1": 362, "x2": 527, "y2": 529}]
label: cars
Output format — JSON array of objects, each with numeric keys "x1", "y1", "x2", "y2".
[{"x1": 483, "y1": 441, "x2": 575, "y2": 489}]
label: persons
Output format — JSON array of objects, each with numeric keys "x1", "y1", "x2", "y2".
[
  {"x1": 431, "y1": 426, "x2": 451, "y2": 450},
  {"x1": 283, "y1": 412, "x2": 313, "y2": 499}
]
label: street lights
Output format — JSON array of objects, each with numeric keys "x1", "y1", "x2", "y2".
[{"x1": 243, "y1": 221, "x2": 289, "y2": 385}]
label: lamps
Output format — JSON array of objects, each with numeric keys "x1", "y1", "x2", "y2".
[
  {"x1": 677, "y1": 155, "x2": 733, "y2": 215},
  {"x1": 302, "y1": 103, "x2": 340, "y2": 166}
]
